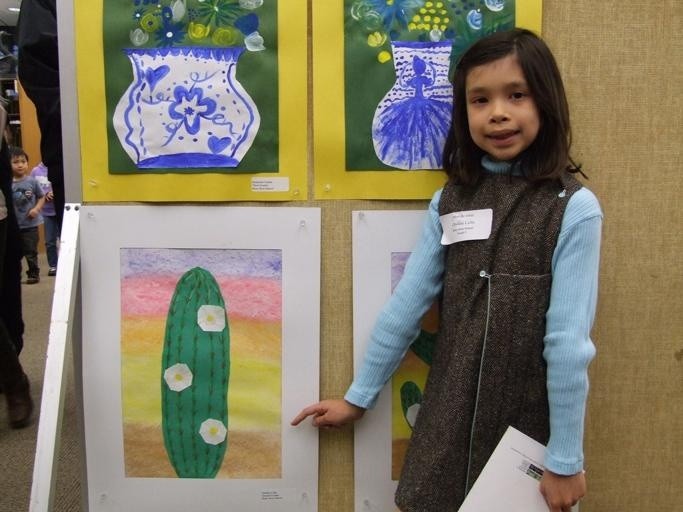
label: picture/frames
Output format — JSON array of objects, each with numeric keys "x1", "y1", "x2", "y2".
[
  {"x1": 350, "y1": 206, "x2": 440, "y2": 512},
  {"x1": 310, "y1": 0, "x2": 544, "y2": 202},
  {"x1": 77, "y1": 202, "x2": 324, "y2": 511},
  {"x1": 70, "y1": 0, "x2": 310, "y2": 203}
]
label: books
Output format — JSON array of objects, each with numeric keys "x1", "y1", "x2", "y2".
[{"x1": 458, "y1": 425, "x2": 585, "y2": 512}]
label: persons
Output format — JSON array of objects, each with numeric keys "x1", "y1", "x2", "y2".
[
  {"x1": 30, "y1": 162, "x2": 61, "y2": 276},
  {"x1": 0, "y1": 96, "x2": 33, "y2": 424},
  {"x1": 290, "y1": 29, "x2": 603, "y2": 512},
  {"x1": 10, "y1": 147, "x2": 46, "y2": 283}
]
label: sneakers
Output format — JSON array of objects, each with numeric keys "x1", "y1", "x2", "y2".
[
  {"x1": 47, "y1": 266, "x2": 57, "y2": 276},
  {"x1": 27, "y1": 276, "x2": 39, "y2": 285}
]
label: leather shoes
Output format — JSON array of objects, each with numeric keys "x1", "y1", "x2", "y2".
[{"x1": 3, "y1": 376, "x2": 35, "y2": 426}]
